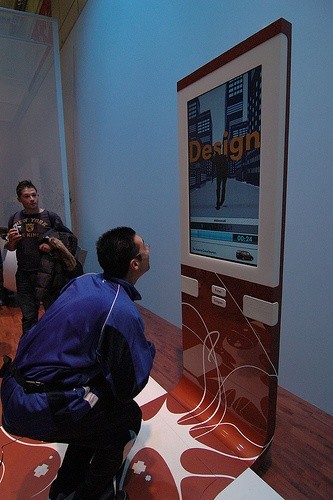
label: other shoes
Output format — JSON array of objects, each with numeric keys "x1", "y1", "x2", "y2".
[
  {"x1": 113, "y1": 490, "x2": 128, "y2": 500},
  {"x1": 47, "y1": 492, "x2": 75, "y2": 500}
]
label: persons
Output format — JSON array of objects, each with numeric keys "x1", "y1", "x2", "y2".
[
  {"x1": 0, "y1": 227, "x2": 156, "y2": 500},
  {"x1": 6, "y1": 181, "x2": 64, "y2": 334}
]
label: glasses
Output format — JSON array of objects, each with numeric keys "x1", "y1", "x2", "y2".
[{"x1": 130, "y1": 243, "x2": 150, "y2": 270}]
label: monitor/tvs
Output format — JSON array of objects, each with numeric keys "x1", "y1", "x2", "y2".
[{"x1": 190, "y1": 64, "x2": 262, "y2": 267}]
